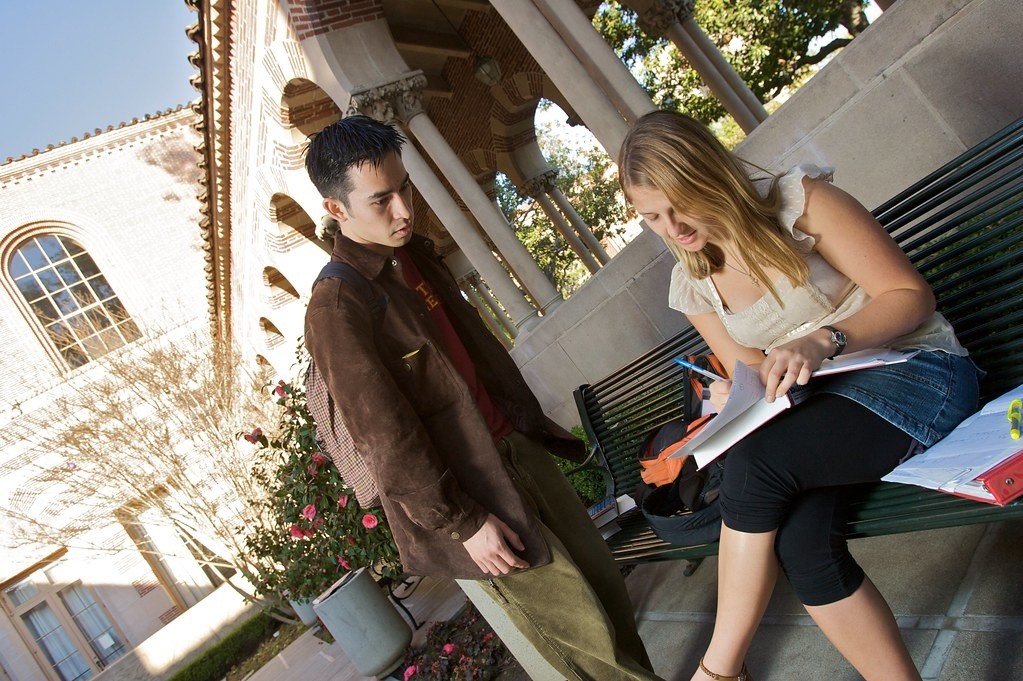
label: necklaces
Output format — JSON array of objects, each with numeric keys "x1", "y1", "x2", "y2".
[{"x1": 715, "y1": 256, "x2": 759, "y2": 287}]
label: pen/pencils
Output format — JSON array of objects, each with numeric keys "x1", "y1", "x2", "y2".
[
  {"x1": 673, "y1": 356, "x2": 728, "y2": 382},
  {"x1": 1008, "y1": 399, "x2": 1022, "y2": 440}
]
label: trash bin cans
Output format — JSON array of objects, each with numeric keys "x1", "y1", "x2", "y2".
[
  {"x1": 313, "y1": 566, "x2": 413, "y2": 679},
  {"x1": 283, "y1": 586, "x2": 317, "y2": 627}
]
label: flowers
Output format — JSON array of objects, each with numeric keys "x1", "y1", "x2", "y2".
[{"x1": 236, "y1": 334, "x2": 413, "y2": 617}]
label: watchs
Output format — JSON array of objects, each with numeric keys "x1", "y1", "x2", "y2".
[{"x1": 819, "y1": 326, "x2": 847, "y2": 360}]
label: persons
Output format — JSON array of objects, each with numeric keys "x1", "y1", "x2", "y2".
[
  {"x1": 618, "y1": 109, "x2": 987, "y2": 681},
  {"x1": 304, "y1": 117, "x2": 663, "y2": 681}
]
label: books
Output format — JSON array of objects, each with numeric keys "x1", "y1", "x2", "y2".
[
  {"x1": 692, "y1": 382, "x2": 814, "y2": 472},
  {"x1": 880, "y1": 446, "x2": 1023, "y2": 507}
]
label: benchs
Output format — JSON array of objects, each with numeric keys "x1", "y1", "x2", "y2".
[
  {"x1": 562, "y1": 118, "x2": 1023, "y2": 578},
  {"x1": 370, "y1": 557, "x2": 426, "y2": 631}
]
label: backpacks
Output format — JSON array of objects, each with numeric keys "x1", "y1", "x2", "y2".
[
  {"x1": 638, "y1": 354, "x2": 727, "y2": 544},
  {"x1": 303, "y1": 263, "x2": 383, "y2": 511}
]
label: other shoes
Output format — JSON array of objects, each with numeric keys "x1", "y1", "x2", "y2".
[{"x1": 688, "y1": 655, "x2": 753, "y2": 681}]
